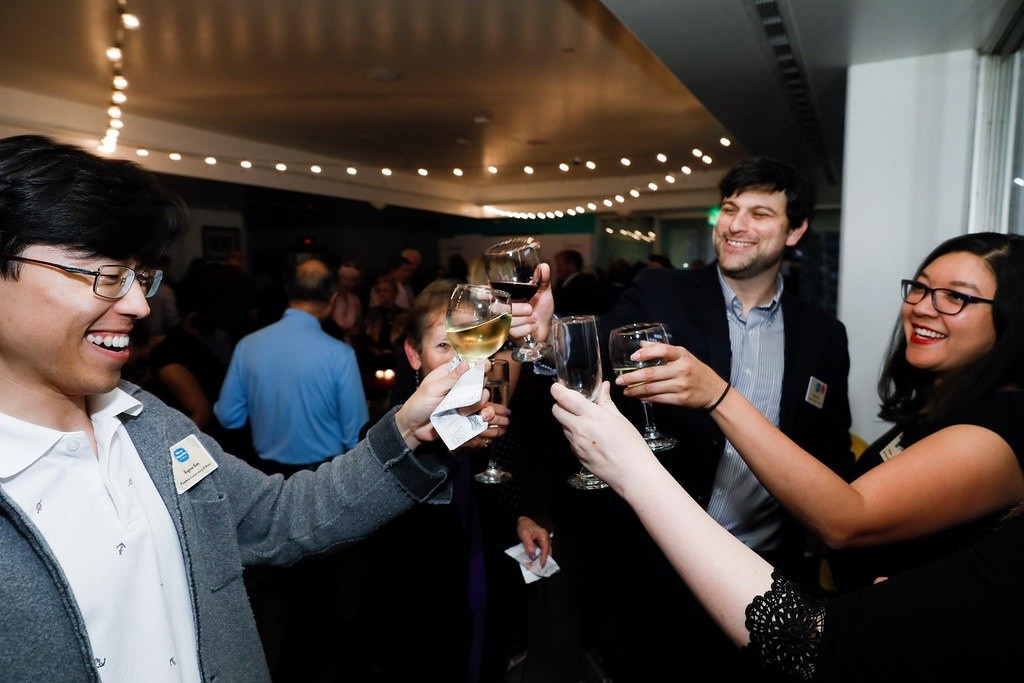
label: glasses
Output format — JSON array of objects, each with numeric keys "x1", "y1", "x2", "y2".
[
  {"x1": 899, "y1": 277, "x2": 994, "y2": 317},
  {"x1": 3, "y1": 254, "x2": 165, "y2": 301}
]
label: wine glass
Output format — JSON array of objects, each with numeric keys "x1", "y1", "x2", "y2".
[
  {"x1": 475, "y1": 359, "x2": 513, "y2": 483},
  {"x1": 553, "y1": 316, "x2": 611, "y2": 491},
  {"x1": 483, "y1": 237, "x2": 552, "y2": 362},
  {"x1": 609, "y1": 323, "x2": 678, "y2": 453}
]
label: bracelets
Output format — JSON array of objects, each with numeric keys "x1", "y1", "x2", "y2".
[{"x1": 704, "y1": 381, "x2": 732, "y2": 414}]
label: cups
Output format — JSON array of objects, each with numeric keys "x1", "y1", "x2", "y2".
[{"x1": 444, "y1": 283, "x2": 511, "y2": 369}]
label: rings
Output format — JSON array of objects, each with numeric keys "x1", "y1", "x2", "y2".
[
  {"x1": 538, "y1": 537, "x2": 548, "y2": 542},
  {"x1": 485, "y1": 438, "x2": 489, "y2": 445}
]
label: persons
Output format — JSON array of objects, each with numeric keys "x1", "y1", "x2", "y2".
[
  {"x1": 551, "y1": 379, "x2": 1024, "y2": 683},
  {"x1": 614, "y1": 232, "x2": 1024, "y2": 648},
  {"x1": 122, "y1": 246, "x2": 671, "y2": 683},
  {"x1": 487, "y1": 149, "x2": 858, "y2": 683},
  {"x1": 0, "y1": 134, "x2": 495, "y2": 683}
]
